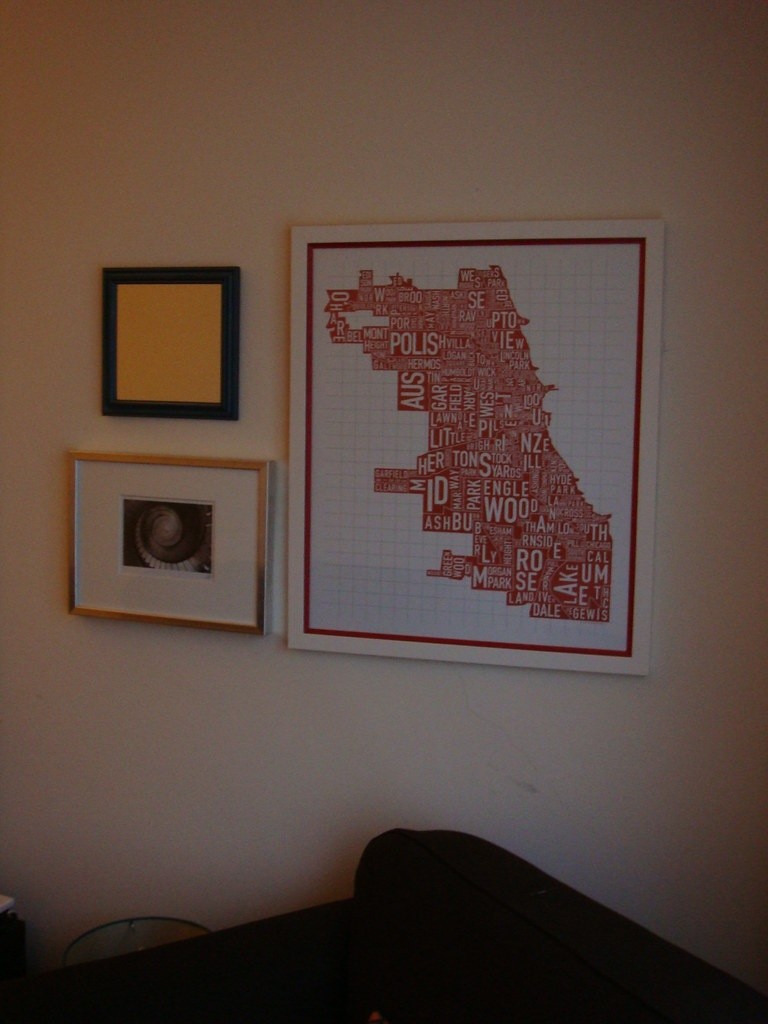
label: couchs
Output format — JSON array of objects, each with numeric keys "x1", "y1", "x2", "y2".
[{"x1": 0, "y1": 829, "x2": 768, "y2": 1024}]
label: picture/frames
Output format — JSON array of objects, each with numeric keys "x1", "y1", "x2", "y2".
[
  {"x1": 68, "y1": 448, "x2": 274, "y2": 636},
  {"x1": 101, "y1": 266, "x2": 241, "y2": 421}
]
